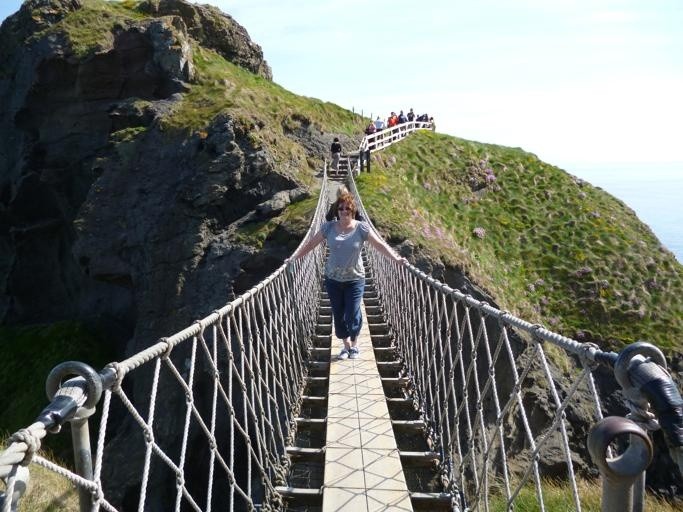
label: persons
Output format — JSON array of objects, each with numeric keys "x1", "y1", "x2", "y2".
[
  {"x1": 330, "y1": 137, "x2": 342, "y2": 177},
  {"x1": 387, "y1": 107, "x2": 435, "y2": 143},
  {"x1": 324, "y1": 186, "x2": 366, "y2": 222},
  {"x1": 367, "y1": 120, "x2": 375, "y2": 150},
  {"x1": 280, "y1": 192, "x2": 407, "y2": 361},
  {"x1": 374, "y1": 115, "x2": 383, "y2": 148}
]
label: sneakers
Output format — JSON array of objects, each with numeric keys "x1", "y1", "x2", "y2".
[
  {"x1": 337, "y1": 347, "x2": 350, "y2": 360},
  {"x1": 347, "y1": 347, "x2": 359, "y2": 359}
]
